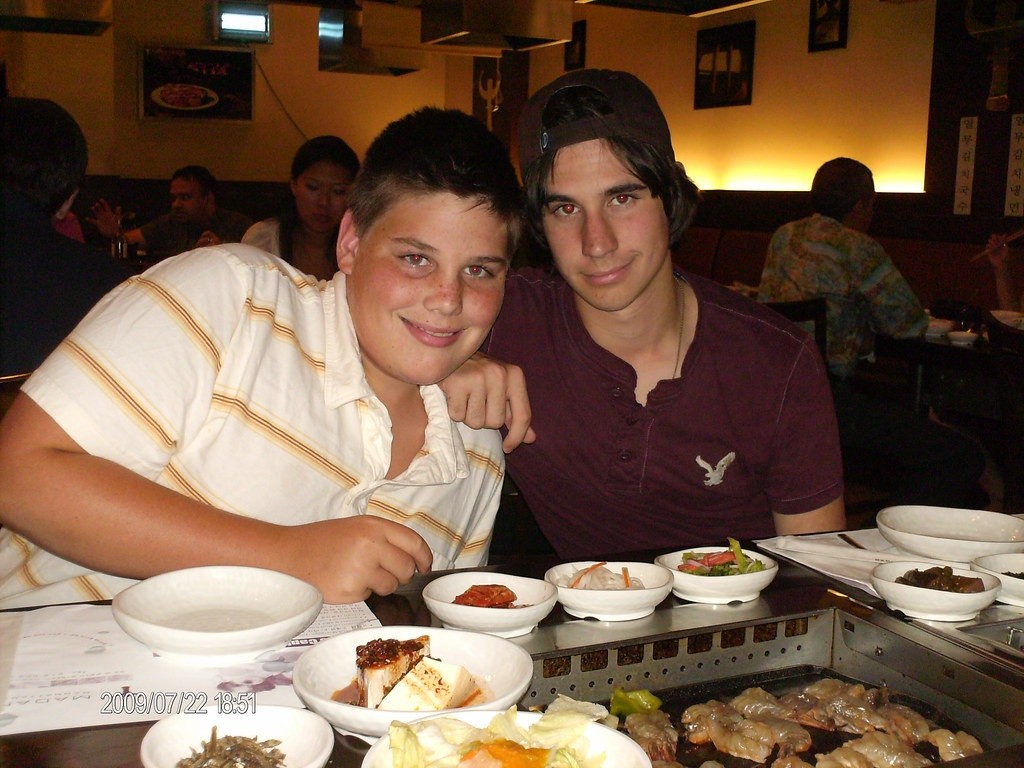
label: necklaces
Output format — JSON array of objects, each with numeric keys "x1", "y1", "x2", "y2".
[{"x1": 672, "y1": 273, "x2": 685, "y2": 378}]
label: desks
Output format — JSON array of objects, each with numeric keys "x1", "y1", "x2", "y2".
[{"x1": 871, "y1": 327, "x2": 1023, "y2": 465}]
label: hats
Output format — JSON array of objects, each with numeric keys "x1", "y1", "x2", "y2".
[{"x1": 518, "y1": 66, "x2": 670, "y2": 171}]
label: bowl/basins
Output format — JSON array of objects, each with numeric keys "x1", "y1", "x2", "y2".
[
  {"x1": 923, "y1": 319, "x2": 956, "y2": 336},
  {"x1": 876, "y1": 505, "x2": 1024, "y2": 565},
  {"x1": 869, "y1": 562, "x2": 1003, "y2": 623},
  {"x1": 545, "y1": 562, "x2": 675, "y2": 621},
  {"x1": 654, "y1": 545, "x2": 779, "y2": 603},
  {"x1": 972, "y1": 551, "x2": 1024, "y2": 605},
  {"x1": 421, "y1": 572, "x2": 558, "y2": 637}
]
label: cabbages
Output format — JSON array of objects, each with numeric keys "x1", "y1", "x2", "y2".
[{"x1": 387, "y1": 694, "x2": 610, "y2": 768}]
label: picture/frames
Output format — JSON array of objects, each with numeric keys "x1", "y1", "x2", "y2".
[
  {"x1": 694, "y1": 19, "x2": 757, "y2": 111},
  {"x1": 808, "y1": 0, "x2": 849, "y2": 53}
]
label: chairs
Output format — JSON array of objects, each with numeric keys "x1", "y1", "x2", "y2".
[{"x1": 754, "y1": 296, "x2": 894, "y2": 483}]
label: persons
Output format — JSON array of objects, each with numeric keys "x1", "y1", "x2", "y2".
[
  {"x1": 0, "y1": 106, "x2": 534, "y2": 620},
  {"x1": 439, "y1": 67, "x2": 842, "y2": 605},
  {"x1": 86, "y1": 164, "x2": 259, "y2": 262},
  {"x1": 0, "y1": 96, "x2": 140, "y2": 378},
  {"x1": 758, "y1": 160, "x2": 998, "y2": 509},
  {"x1": 983, "y1": 233, "x2": 1024, "y2": 335},
  {"x1": 242, "y1": 135, "x2": 361, "y2": 284}
]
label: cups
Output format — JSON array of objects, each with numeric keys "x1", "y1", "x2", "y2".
[{"x1": 110, "y1": 215, "x2": 128, "y2": 261}]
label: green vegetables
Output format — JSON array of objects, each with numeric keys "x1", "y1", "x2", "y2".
[
  {"x1": 676, "y1": 537, "x2": 768, "y2": 577},
  {"x1": 607, "y1": 687, "x2": 664, "y2": 716}
]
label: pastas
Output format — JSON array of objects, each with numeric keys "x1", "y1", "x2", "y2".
[{"x1": 562, "y1": 565, "x2": 645, "y2": 589}]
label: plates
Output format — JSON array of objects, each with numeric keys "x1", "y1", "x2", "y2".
[
  {"x1": 139, "y1": 703, "x2": 335, "y2": 768},
  {"x1": 292, "y1": 625, "x2": 532, "y2": 734},
  {"x1": 110, "y1": 564, "x2": 323, "y2": 650},
  {"x1": 150, "y1": 83, "x2": 218, "y2": 110},
  {"x1": 361, "y1": 706, "x2": 653, "y2": 768}
]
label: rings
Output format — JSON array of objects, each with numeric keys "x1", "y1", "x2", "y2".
[{"x1": 208, "y1": 237, "x2": 212, "y2": 242}]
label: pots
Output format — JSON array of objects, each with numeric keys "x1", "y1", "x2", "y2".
[{"x1": 948, "y1": 331, "x2": 979, "y2": 345}]
